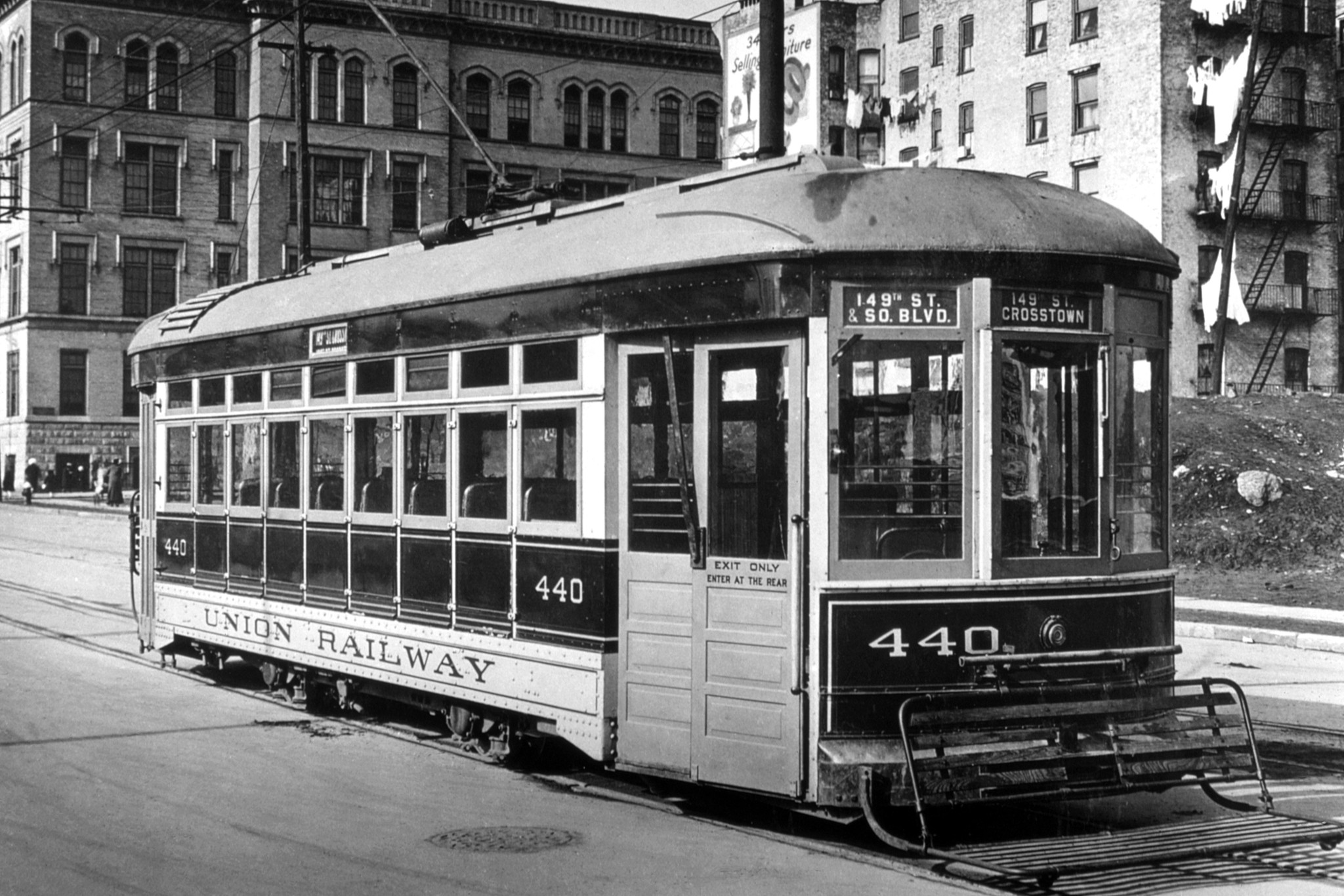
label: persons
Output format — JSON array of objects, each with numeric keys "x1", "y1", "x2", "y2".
[
  {"x1": 43, "y1": 463, "x2": 56, "y2": 493},
  {"x1": 61, "y1": 463, "x2": 72, "y2": 492},
  {"x1": 106, "y1": 464, "x2": 125, "y2": 506},
  {"x1": 25, "y1": 458, "x2": 42, "y2": 491},
  {"x1": 93, "y1": 463, "x2": 108, "y2": 500}
]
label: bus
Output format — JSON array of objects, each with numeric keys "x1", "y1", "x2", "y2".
[{"x1": 131, "y1": 1, "x2": 1344, "y2": 886}]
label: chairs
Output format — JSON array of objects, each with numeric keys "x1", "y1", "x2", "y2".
[{"x1": 237, "y1": 474, "x2": 575, "y2": 521}]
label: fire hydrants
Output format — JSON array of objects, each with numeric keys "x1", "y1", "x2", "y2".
[{"x1": 21, "y1": 481, "x2": 31, "y2": 505}]
